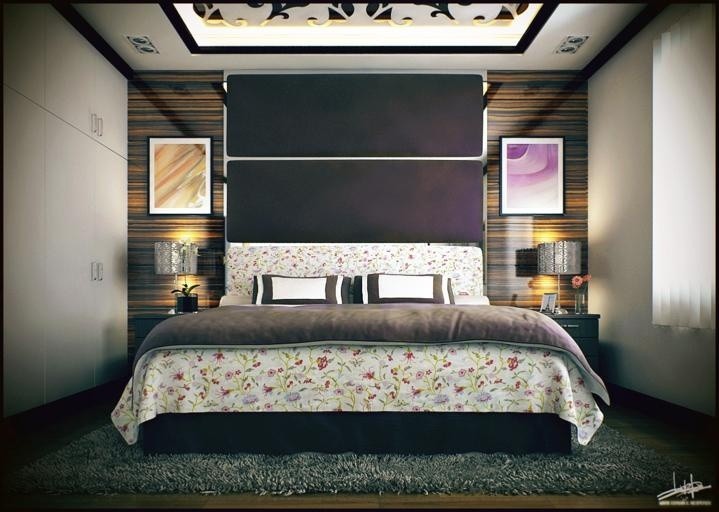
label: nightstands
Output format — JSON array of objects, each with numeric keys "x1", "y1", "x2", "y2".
[
  {"x1": 538, "y1": 311, "x2": 600, "y2": 374},
  {"x1": 133, "y1": 313, "x2": 169, "y2": 356}
]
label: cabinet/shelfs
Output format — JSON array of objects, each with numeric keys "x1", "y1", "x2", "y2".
[{"x1": 2, "y1": 2, "x2": 128, "y2": 417}]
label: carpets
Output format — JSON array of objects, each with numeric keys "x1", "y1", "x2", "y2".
[{"x1": 7, "y1": 423, "x2": 712, "y2": 495}]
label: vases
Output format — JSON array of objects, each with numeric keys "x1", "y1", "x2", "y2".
[
  {"x1": 574, "y1": 293, "x2": 587, "y2": 315},
  {"x1": 176, "y1": 297, "x2": 198, "y2": 313}
]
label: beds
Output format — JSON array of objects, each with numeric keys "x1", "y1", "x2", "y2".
[{"x1": 110, "y1": 243, "x2": 610, "y2": 455}]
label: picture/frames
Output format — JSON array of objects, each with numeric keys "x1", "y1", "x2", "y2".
[
  {"x1": 499, "y1": 136, "x2": 565, "y2": 219},
  {"x1": 148, "y1": 135, "x2": 213, "y2": 216},
  {"x1": 540, "y1": 292, "x2": 557, "y2": 313}
]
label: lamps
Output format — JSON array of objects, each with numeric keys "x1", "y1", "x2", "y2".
[
  {"x1": 154, "y1": 240, "x2": 196, "y2": 315},
  {"x1": 538, "y1": 242, "x2": 581, "y2": 313}
]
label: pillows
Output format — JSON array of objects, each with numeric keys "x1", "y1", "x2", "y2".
[{"x1": 252, "y1": 273, "x2": 455, "y2": 306}]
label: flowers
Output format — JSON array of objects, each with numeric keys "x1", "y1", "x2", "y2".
[
  {"x1": 571, "y1": 274, "x2": 592, "y2": 311},
  {"x1": 171, "y1": 245, "x2": 199, "y2": 297}
]
